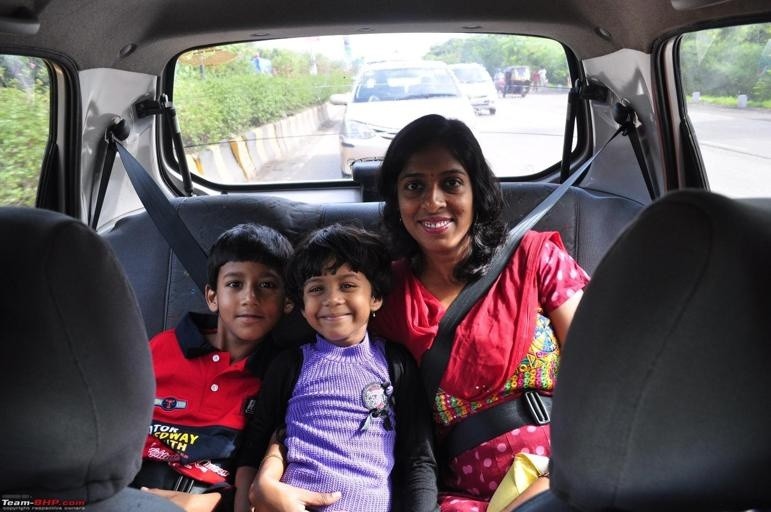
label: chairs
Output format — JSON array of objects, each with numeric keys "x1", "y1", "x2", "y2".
[{"x1": 1, "y1": 182, "x2": 771, "y2": 511}]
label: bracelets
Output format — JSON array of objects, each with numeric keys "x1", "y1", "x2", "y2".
[{"x1": 257, "y1": 454, "x2": 284, "y2": 466}]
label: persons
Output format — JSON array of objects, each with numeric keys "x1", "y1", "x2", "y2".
[
  {"x1": 123, "y1": 221, "x2": 295, "y2": 512},
  {"x1": 232, "y1": 223, "x2": 439, "y2": 512},
  {"x1": 492, "y1": 67, "x2": 507, "y2": 98},
  {"x1": 249, "y1": 114, "x2": 592, "y2": 512},
  {"x1": 530, "y1": 63, "x2": 548, "y2": 94}
]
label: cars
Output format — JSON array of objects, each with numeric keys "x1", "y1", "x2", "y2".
[{"x1": 330, "y1": 58, "x2": 530, "y2": 178}]
